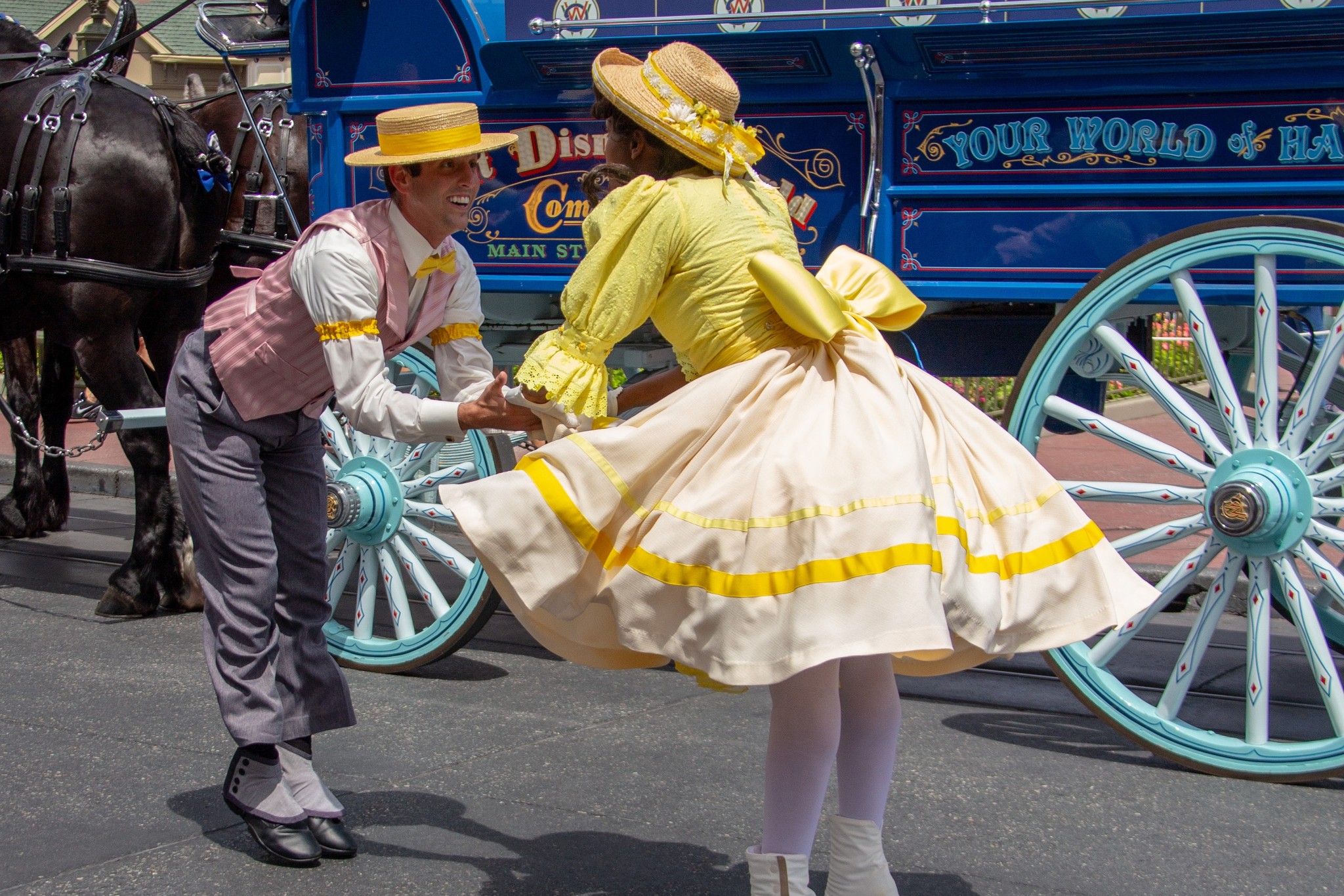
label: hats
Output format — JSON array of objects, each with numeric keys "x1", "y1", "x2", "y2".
[
  {"x1": 591, "y1": 42, "x2": 782, "y2": 202},
  {"x1": 343, "y1": 103, "x2": 520, "y2": 166}
]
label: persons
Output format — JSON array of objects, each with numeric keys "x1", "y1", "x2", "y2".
[
  {"x1": 438, "y1": 43, "x2": 1161, "y2": 896},
  {"x1": 70, "y1": 337, "x2": 155, "y2": 419},
  {"x1": 165, "y1": 102, "x2": 542, "y2": 863}
]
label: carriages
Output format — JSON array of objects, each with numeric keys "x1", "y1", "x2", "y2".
[{"x1": 0, "y1": 1, "x2": 1343, "y2": 787}]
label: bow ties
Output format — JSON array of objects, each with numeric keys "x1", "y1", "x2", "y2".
[{"x1": 416, "y1": 249, "x2": 456, "y2": 279}]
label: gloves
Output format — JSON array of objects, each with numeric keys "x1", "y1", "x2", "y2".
[{"x1": 574, "y1": 386, "x2": 624, "y2": 432}]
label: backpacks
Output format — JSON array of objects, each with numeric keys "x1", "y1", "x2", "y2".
[{"x1": 504, "y1": 382, "x2": 579, "y2": 428}]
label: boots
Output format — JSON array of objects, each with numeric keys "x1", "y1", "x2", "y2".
[
  {"x1": 746, "y1": 843, "x2": 820, "y2": 896},
  {"x1": 823, "y1": 812, "x2": 899, "y2": 896}
]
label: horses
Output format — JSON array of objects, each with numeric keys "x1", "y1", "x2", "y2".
[{"x1": 0, "y1": 17, "x2": 306, "y2": 619}]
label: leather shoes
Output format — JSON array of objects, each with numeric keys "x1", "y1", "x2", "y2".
[
  {"x1": 223, "y1": 794, "x2": 322, "y2": 864},
  {"x1": 306, "y1": 815, "x2": 360, "y2": 857}
]
